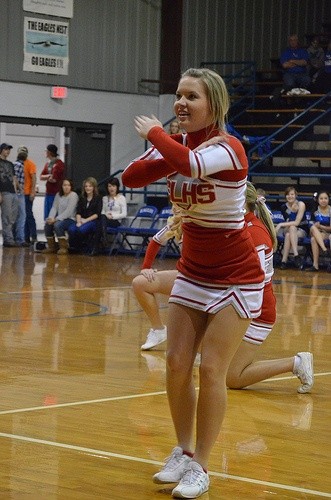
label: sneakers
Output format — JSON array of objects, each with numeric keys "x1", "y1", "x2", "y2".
[
  {"x1": 141, "y1": 352, "x2": 167, "y2": 372},
  {"x1": 294, "y1": 395, "x2": 312, "y2": 431},
  {"x1": 172, "y1": 461, "x2": 209, "y2": 497},
  {"x1": 153, "y1": 447, "x2": 195, "y2": 483},
  {"x1": 140, "y1": 324, "x2": 168, "y2": 350},
  {"x1": 294, "y1": 351, "x2": 314, "y2": 394}
]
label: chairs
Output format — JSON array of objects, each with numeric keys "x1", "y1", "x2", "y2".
[
  {"x1": 269, "y1": 210, "x2": 331, "y2": 273},
  {"x1": 137, "y1": 207, "x2": 181, "y2": 262},
  {"x1": 89, "y1": 205, "x2": 136, "y2": 257},
  {"x1": 114, "y1": 206, "x2": 160, "y2": 258}
]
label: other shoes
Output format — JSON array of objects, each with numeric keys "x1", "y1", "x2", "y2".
[
  {"x1": 299, "y1": 88, "x2": 311, "y2": 94},
  {"x1": 306, "y1": 266, "x2": 319, "y2": 273},
  {"x1": 294, "y1": 255, "x2": 302, "y2": 266},
  {"x1": 287, "y1": 89, "x2": 301, "y2": 95},
  {"x1": 324, "y1": 251, "x2": 329, "y2": 258},
  {"x1": 22, "y1": 242, "x2": 31, "y2": 247},
  {"x1": 279, "y1": 262, "x2": 288, "y2": 269}
]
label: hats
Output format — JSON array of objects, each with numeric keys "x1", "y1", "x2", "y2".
[
  {"x1": 47, "y1": 144, "x2": 59, "y2": 155},
  {"x1": 0, "y1": 143, "x2": 13, "y2": 149}
]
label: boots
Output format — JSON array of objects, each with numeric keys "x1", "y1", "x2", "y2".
[
  {"x1": 58, "y1": 238, "x2": 68, "y2": 253},
  {"x1": 43, "y1": 236, "x2": 58, "y2": 252}
]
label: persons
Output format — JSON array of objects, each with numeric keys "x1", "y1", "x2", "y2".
[
  {"x1": 13, "y1": 153, "x2": 32, "y2": 247},
  {"x1": 275, "y1": 187, "x2": 308, "y2": 270},
  {"x1": 279, "y1": 32, "x2": 311, "y2": 93},
  {"x1": 89, "y1": 178, "x2": 127, "y2": 256},
  {"x1": 69, "y1": 177, "x2": 102, "y2": 256},
  {"x1": 226, "y1": 181, "x2": 314, "y2": 394},
  {"x1": 308, "y1": 192, "x2": 331, "y2": 272},
  {"x1": 308, "y1": 37, "x2": 325, "y2": 71},
  {"x1": 0, "y1": 143, "x2": 19, "y2": 248},
  {"x1": 42, "y1": 178, "x2": 79, "y2": 254},
  {"x1": 17, "y1": 146, "x2": 37, "y2": 246},
  {"x1": 40, "y1": 144, "x2": 66, "y2": 220},
  {"x1": 132, "y1": 202, "x2": 184, "y2": 350},
  {"x1": 122, "y1": 67, "x2": 265, "y2": 499}
]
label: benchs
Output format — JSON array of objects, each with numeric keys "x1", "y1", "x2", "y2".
[{"x1": 146, "y1": 21, "x2": 331, "y2": 214}]
